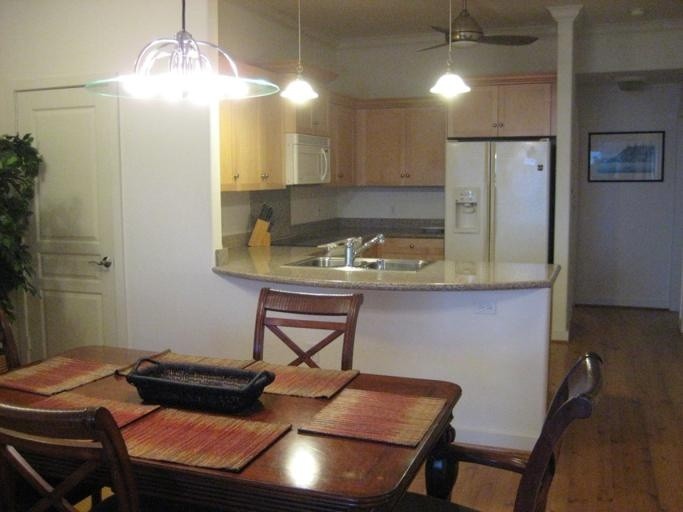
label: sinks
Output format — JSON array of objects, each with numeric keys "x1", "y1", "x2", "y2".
[
  {"x1": 279, "y1": 256, "x2": 368, "y2": 270},
  {"x1": 368, "y1": 259, "x2": 440, "y2": 273}
]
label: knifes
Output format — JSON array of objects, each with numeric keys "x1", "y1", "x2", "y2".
[{"x1": 257, "y1": 203, "x2": 273, "y2": 222}]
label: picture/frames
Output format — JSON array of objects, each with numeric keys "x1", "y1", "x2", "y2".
[{"x1": 583, "y1": 128, "x2": 667, "y2": 185}]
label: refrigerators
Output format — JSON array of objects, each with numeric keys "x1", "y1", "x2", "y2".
[{"x1": 444, "y1": 139, "x2": 550, "y2": 264}]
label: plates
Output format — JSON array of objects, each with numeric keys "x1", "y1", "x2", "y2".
[{"x1": 420, "y1": 226, "x2": 443, "y2": 232}]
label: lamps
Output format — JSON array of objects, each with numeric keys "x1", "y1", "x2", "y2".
[
  {"x1": 274, "y1": 0, "x2": 320, "y2": 108},
  {"x1": 444, "y1": 29, "x2": 485, "y2": 50},
  {"x1": 428, "y1": 0, "x2": 472, "y2": 101},
  {"x1": 77, "y1": 0, "x2": 280, "y2": 116}
]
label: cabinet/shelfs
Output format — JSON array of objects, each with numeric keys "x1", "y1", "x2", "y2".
[
  {"x1": 278, "y1": 71, "x2": 331, "y2": 137},
  {"x1": 444, "y1": 74, "x2": 558, "y2": 142},
  {"x1": 214, "y1": 57, "x2": 286, "y2": 196},
  {"x1": 353, "y1": 99, "x2": 446, "y2": 191},
  {"x1": 319, "y1": 93, "x2": 357, "y2": 190}
]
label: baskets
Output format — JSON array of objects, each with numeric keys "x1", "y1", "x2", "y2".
[{"x1": 126, "y1": 357, "x2": 275, "y2": 411}]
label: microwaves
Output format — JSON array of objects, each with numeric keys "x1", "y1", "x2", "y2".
[{"x1": 284, "y1": 132, "x2": 331, "y2": 187}]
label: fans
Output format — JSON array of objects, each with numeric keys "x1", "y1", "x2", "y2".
[{"x1": 410, "y1": 0, "x2": 538, "y2": 58}]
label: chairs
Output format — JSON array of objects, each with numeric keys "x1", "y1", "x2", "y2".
[
  {"x1": 246, "y1": 283, "x2": 364, "y2": 372},
  {"x1": 0, "y1": 308, "x2": 20, "y2": 379},
  {"x1": 0, "y1": 400, "x2": 141, "y2": 512},
  {"x1": 390, "y1": 350, "x2": 606, "y2": 511}
]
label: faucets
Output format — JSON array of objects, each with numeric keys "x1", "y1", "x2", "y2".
[{"x1": 346, "y1": 234, "x2": 386, "y2": 266}]
